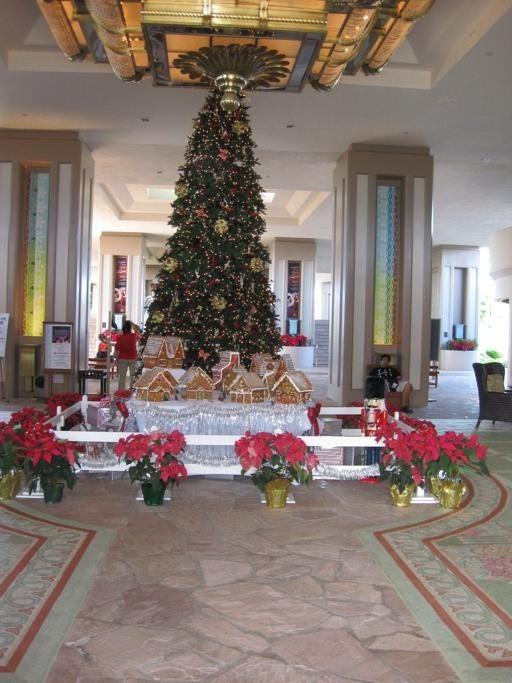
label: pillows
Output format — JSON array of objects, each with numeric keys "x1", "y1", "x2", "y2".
[{"x1": 487, "y1": 375, "x2": 505, "y2": 392}]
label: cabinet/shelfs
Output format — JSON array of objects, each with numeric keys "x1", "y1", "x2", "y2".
[{"x1": 80, "y1": 369, "x2": 107, "y2": 395}]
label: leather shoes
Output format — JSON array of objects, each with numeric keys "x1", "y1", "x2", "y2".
[{"x1": 400, "y1": 407, "x2": 414, "y2": 414}]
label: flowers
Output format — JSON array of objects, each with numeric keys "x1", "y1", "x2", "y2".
[
  {"x1": 235, "y1": 430, "x2": 320, "y2": 493},
  {"x1": 350, "y1": 400, "x2": 490, "y2": 510},
  {"x1": 282, "y1": 334, "x2": 307, "y2": 346},
  {"x1": 113, "y1": 429, "x2": 188, "y2": 491},
  {"x1": 0, "y1": 393, "x2": 105, "y2": 503},
  {"x1": 443, "y1": 337, "x2": 477, "y2": 351}
]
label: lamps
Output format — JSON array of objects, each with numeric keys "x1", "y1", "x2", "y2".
[{"x1": 36, "y1": 0, "x2": 437, "y2": 115}]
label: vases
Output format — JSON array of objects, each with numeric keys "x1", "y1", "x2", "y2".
[
  {"x1": 141, "y1": 482, "x2": 166, "y2": 506},
  {"x1": 264, "y1": 478, "x2": 290, "y2": 508}
]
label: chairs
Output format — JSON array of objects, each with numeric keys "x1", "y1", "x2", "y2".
[
  {"x1": 472, "y1": 362, "x2": 511, "y2": 428},
  {"x1": 364, "y1": 364, "x2": 413, "y2": 410},
  {"x1": 429, "y1": 366, "x2": 439, "y2": 388}
]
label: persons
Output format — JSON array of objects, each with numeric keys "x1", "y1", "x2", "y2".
[
  {"x1": 369, "y1": 354, "x2": 414, "y2": 414},
  {"x1": 95, "y1": 320, "x2": 141, "y2": 392}
]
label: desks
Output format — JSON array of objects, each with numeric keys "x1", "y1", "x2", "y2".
[{"x1": 89, "y1": 357, "x2": 118, "y2": 379}]
